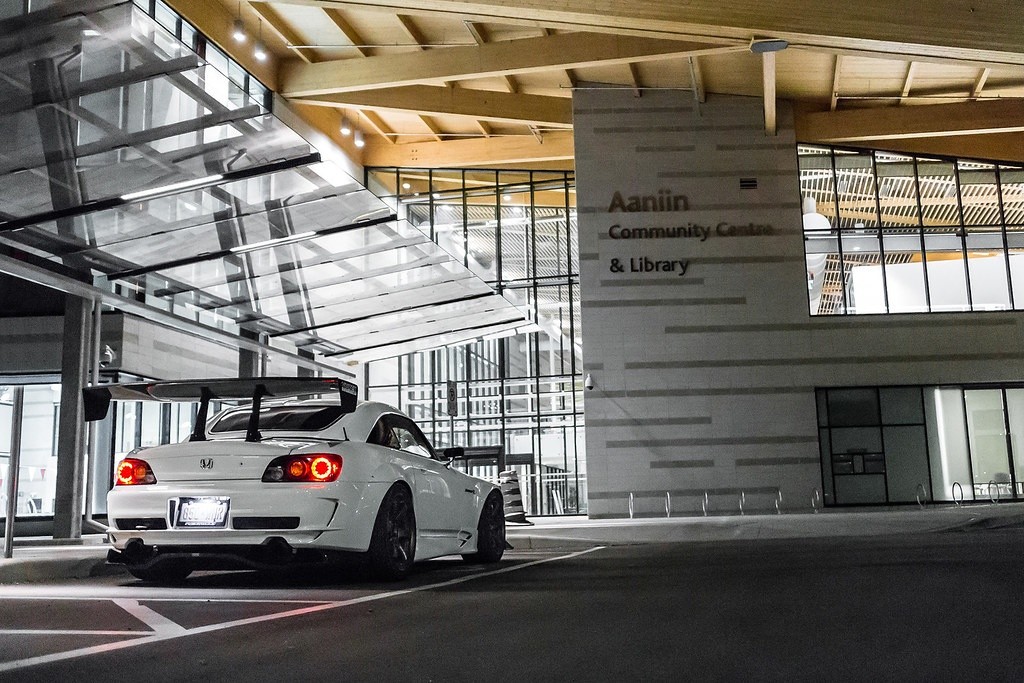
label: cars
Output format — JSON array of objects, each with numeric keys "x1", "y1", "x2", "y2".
[{"x1": 82, "y1": 372, "x2": 514, "y2": 582}]
